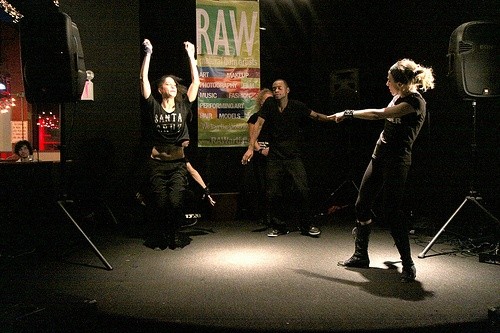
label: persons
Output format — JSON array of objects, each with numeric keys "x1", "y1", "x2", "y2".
[
  {"x1": 246, "y1": 88, "x2": 273, "y2": 225},
  {"x1": 140, "y1": 38, "x2": 216, "y2": 250},
  {"x1": 241, "y1": 59, "x2": 336, "y2": 238},
  {"x1": 326, "y1": 59, "x2": 434, "y2": 283},
  {"x1": 14, "y1": 140, "x2": 33, "y2": 162}
]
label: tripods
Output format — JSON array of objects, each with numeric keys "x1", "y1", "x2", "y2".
[{"x1": 418, "y1": 102, "x2": 500, "y2": 259}]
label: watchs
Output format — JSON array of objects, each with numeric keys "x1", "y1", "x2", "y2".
[{"x1": 258, "y1": 148, "x2": 262, "y2": 154}]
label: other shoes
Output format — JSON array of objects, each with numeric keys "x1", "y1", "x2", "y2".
[
  {"x1": 337, "y1": 258, "x2": 369, "y2": 268},
  {"x1": 307, "y1": 226, "x2": 321, "y2": 236},
  {"x1": 267, "y1": 229, "x2": 289, "y2": 237},
  {"x1": 399, "y1": 266, "x2": 415, "y2": 282}
]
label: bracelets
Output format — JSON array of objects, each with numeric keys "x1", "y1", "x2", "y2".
[{"x1": 344, "y1": 109, "x2": 354, "y2": 120}]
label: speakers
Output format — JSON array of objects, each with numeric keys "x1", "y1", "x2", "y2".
[
  {"x1": 18, "y1": 10, "x2": 86, "y2": 105},
  {"x1": 330, "y1": 68, "x2": 360, "y2": 114},
  {"x1": 447, "y1": 21, "x2": 500, "y2": 103}
]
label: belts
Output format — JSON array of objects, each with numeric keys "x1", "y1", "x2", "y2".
[{"x1": 149, "y1": 155, "x2": 186, "y2": 163}]
